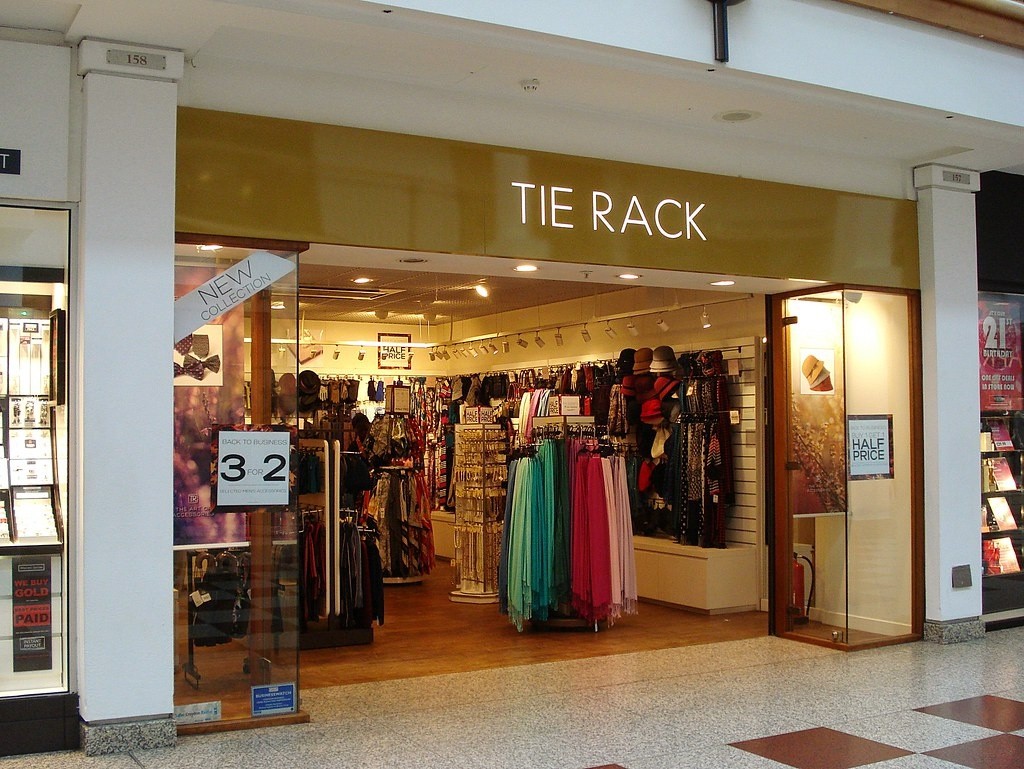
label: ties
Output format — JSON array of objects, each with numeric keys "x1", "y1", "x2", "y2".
[
  {"x1": 192, "y1": 334, "x2": 209, "y2": 358},
  {"x1": 175, "y1": 334, "x2": 192, "y2": 356}
]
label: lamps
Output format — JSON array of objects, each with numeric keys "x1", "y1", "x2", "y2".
[
  {"x1": 429, "y1": 303, "x2": 711, "y2": 362},
  {"x1": 280, "y1": 346, "x2": 366, "y2": 360}
]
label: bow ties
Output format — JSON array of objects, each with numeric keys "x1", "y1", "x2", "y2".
[
  {"x1": 173, "y1": 362, "x2": 203, "y2": 381},
  {"x1": 183, "y1": 354, "x2": 220, "y2": 373}
]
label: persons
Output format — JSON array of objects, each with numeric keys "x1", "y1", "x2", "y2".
[{"x1": 342, "y1": 413, "x2": 392, "y2": 507}]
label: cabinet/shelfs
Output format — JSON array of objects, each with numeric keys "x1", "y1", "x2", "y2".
[
  {"x1": 450, "y1": 406, "x2": 508, "y2": 605},
  {"x1": 298, "y1": 436, "x2": 429, "y2": 648}
]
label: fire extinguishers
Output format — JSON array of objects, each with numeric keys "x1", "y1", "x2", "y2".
[{"x1": 792, "y1": 552, "x2": 815, "y2": 625}]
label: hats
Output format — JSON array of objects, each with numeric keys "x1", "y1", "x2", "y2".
[
  {"x1": 617, "y1": 348, "x2": 636, "y2": 368},
  {"x1": 637, "y1": 376, "x2": 660, "y2": 400},
  {"x1": 802, "y1": 355, "x2": 833, "y2": 391},
  {"x1": 641, "y1": 399, "x2": 662, "y2": 417},
  {"x1": 650, "y1": 345, "x2": 678, "y2": 372},
  {"x1": 632, "y1": 347, "x2": 654, "y2": 375},
  {"x1": 620, "y1": 376, "x2": 636, "y2": 396},
  {"x1": 298, "y1": 370, "x2": 321, "y2": 394},
  {"x1": 299, "y1": 392, "x2": 320, "y2": 412},
  {"x1": 653, "y1": 377, "x2": 681, "y2": 401}
]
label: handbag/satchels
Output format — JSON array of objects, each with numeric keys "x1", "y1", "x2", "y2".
[{"x1": 502, "y1": 369, "x2": 538, "y2": 419}]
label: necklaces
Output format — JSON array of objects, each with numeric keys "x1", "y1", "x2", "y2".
[{"x1": 451, "y1": 488, "x2": 505, "y2": 594}]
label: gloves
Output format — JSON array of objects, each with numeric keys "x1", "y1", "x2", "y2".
[
  {"x1": 340, "y1": 380, "x2": 351, "y2": 400},
  {"x1": 349, "y1": 380, "x2": 359, "y2": 401},
  {"x1": 319, "y1": 379, "x2": 328, "y2": 401},
  {"x1": 330, "y1": 381, "x2": 340, "y2": 403}
]
